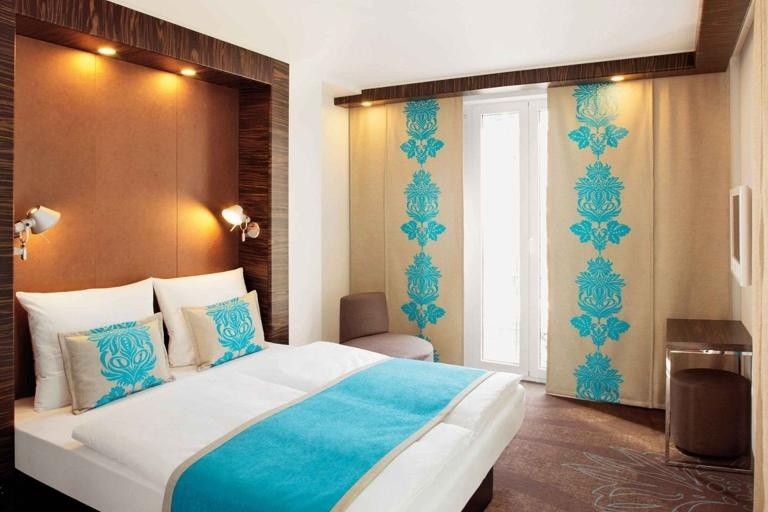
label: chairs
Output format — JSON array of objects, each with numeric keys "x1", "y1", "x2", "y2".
[{"x1": 339, "y1": 292, "x2": 433, "y2": 361}]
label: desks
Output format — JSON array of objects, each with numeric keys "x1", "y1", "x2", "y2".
[{"x1": 664, "y1": 318, "x2": 752, "y2": 476}]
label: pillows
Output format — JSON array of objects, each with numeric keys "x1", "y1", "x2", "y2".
[
  {"x1": 18, "y1": 275, "x2": 159, "y2": 412},
  {"x1": 152, "y1": 268, "x2": 250, "y2": 367},
  {"x1": 180, "y1": 291, "x2": 269, "y2": 368},
  {"x1": 59, "y1": 312, "x2": 171, "y2": 412}
]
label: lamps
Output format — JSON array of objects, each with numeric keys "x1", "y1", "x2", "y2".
[
  {"x1": 14, "y1": 202, "x2": 62, "y2": 258},
  {"x1": 222, "y1": 205, "x2": 260, "y2": 243}
]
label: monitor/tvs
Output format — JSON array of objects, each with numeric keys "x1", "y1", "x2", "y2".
[{"x1": 728, "y1": 185, "x2": 751, "y2": 287}]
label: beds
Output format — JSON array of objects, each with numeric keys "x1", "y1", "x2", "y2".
[{"x1": 15, "y1": 341, "x2": 523, "y2": 512}]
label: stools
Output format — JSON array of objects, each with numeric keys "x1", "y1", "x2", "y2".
[{"x1": 671, "y1": 367, "x2": 751, "y2": 459}]
